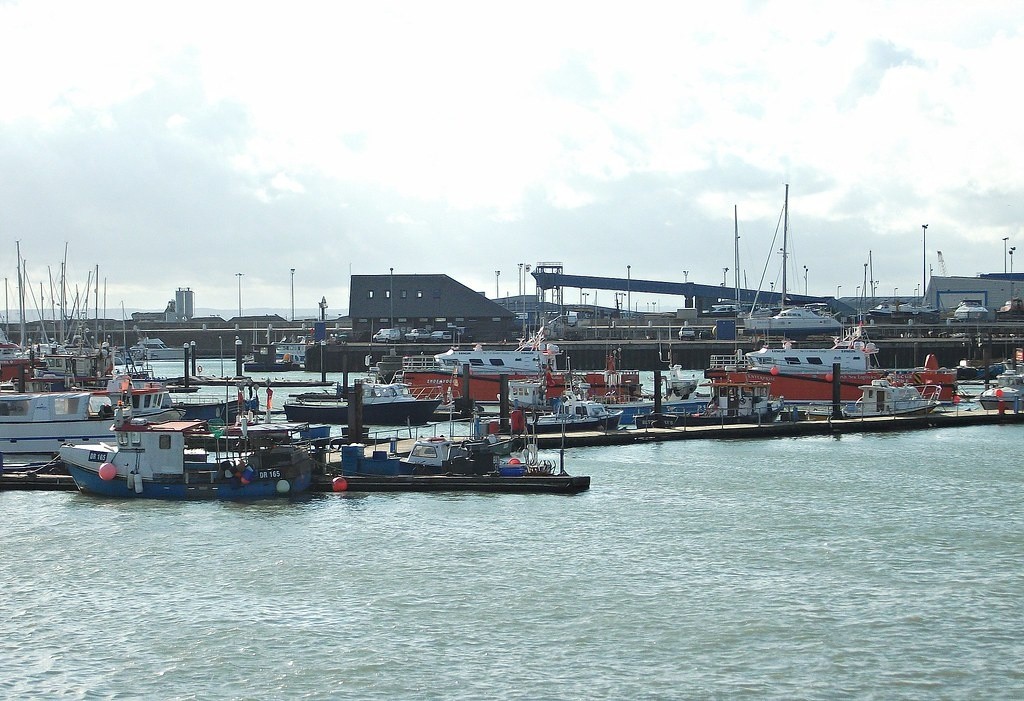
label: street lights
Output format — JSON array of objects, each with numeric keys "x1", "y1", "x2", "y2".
[
  {"x1": 836, "y1": 285, "x2": 842, "y2": 298},
  {"x1": 523, "y1": 264, "x2": 532, "y2": 343},
  {"x1": 390, "y1": 267, "x2": 395, "y2": 330},
  {"x1": 855, "y1": 285, "x2": 861, "y2": 297},
  {"x1": 921, "y1": 223, "x2": 928, "y2": 297},
  {"x1": 517, "y1": 263, "x2": 524, "y2": 297},
  {"x1": 1009, "y1": 246, "x2": 1017, "y2": 273},
  {"x1": 627, "y1": 264, "x2": 631, "y2": 327},
  {"x1": 914, "y1": 288, "x2": 917, "y2": 295},
  {"x1": 1003, "y1": 237, "x2": 1010, "y2": 272},
  {"x1": 723, "y1": 267, "x2": 729, "y2": 288},
  {"x1": 234, "y1": 272, "x2": 245, "y2": 319},
  {"x1": 917, "y1": 283, "x2": 921, "y2": 297},
  {"x1": 682, "y1": 270, "x2": 689, "y2": 282},
  {"x1": 893, "y1": 287, "x2": 898, "y2": 296},
  {"x1": 864, "y1": 263, "x2": 868, "y2": 311},
  {"x1": 803, "y1": 265, "x2": 809, "y2": 297},
  {"x1": 290, "y1": 268, "x2": 296, "y2": 320},
  {"x1": 495, "y1": 271, "x2": 501, "y2": 298}
]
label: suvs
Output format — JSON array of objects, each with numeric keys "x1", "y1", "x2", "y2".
[
  {"x1": 431, "y1": 331, "x2": 452, "y2": 342},
  {"x1": 404, "y1": 328, "x2": 431, "y2": 344},
  {"x1": 373, "y1": 329, "x2": 401, "y2": 344}
]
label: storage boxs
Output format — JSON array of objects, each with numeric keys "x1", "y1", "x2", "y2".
[
  {"x1": 499, "y1": 464, "x2": 528, "y2": 477},
  {"x1": 300, "y1": 424, "x2": 331, "y2": 441},
  {"x1": 341, "y1": 427, "x2": 369, "y2": 438}
]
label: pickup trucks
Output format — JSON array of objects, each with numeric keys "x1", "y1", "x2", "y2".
[{"x1": 679, "y1": 326, "x2": 696, "y2": 341}]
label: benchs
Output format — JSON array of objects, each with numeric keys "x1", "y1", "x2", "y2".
[{"x1": 184, "y1": 470, "x2": 218, "y2": 483}]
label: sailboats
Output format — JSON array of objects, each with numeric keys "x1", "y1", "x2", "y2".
[{"x1": 744, "y1": 183, "x2": 847, "y2": 341}]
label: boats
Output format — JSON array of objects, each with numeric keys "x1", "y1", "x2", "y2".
[{"x1": 0, "y1": 239, "x2": 1024, "y2": 495}]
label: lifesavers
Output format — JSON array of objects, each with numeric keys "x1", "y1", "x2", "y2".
[
  {"x1": 424, "y1": 437, "x2": 445, "y2": 440},
  {"x1": 198, "y1": 366, "x2": 202, "y2": 372}
]
